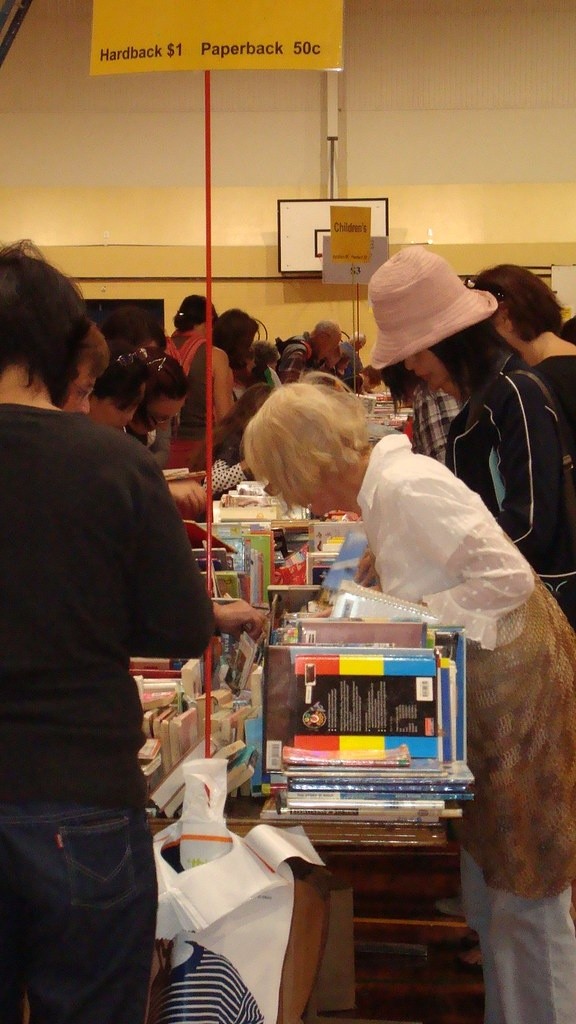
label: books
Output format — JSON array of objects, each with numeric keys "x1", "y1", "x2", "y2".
[
  {"x1": 347, "y1": 387, "x2": 418, "y2": 448},
  {"x1": 128, "y1": 482, "x2": 475, "y2": 822}
]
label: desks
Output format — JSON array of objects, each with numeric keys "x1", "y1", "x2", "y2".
[{"x1": 149, "y1": 797, "x2": 485, "y2": 1024}]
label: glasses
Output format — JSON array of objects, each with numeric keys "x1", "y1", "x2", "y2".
[
  {"x1": 71, "y1": 378, "x2": 93, "y2": 401},
  {"x1": 138, "y1": 406, "x2": 182, "y2": 429}
]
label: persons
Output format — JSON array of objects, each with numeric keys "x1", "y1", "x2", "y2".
[{"x1": 0, "y1": 239, "x2": 576, "y2": 1024}]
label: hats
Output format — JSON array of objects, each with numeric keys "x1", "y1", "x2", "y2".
[{"x1": 368, "y1": 245, "x2": 498, "y2": 370}]
